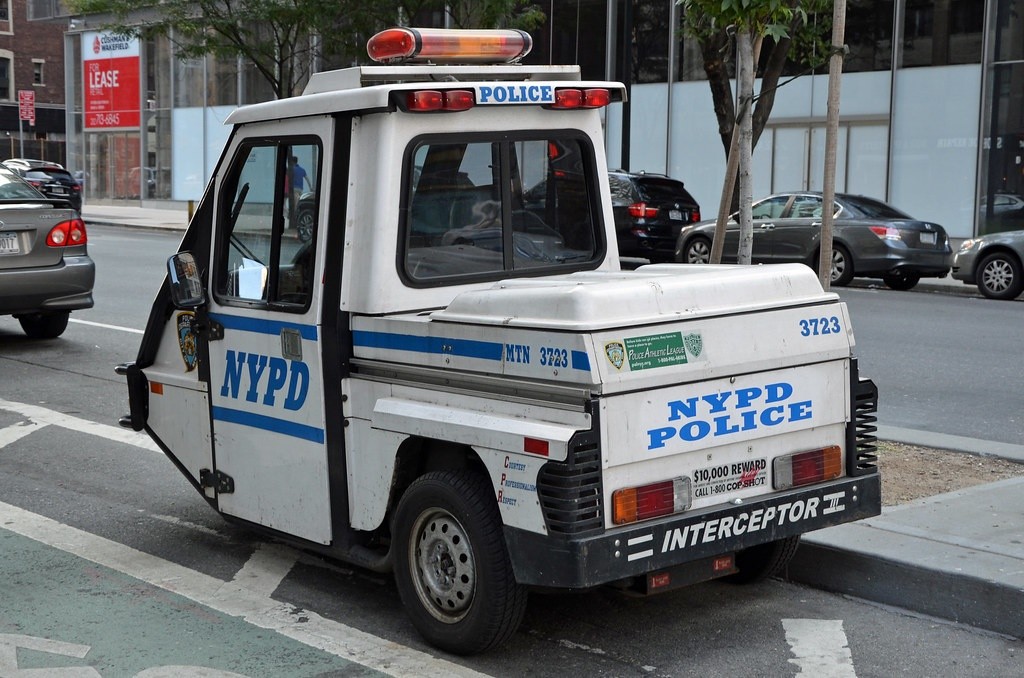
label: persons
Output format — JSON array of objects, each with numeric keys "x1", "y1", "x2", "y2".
[{"x1": 284, "y1": 155, "x2": 316, "y2": 230}]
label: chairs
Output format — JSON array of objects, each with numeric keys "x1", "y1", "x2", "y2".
[{"x1": 799, "y1": 203, "x2": 814, "y2": 216}]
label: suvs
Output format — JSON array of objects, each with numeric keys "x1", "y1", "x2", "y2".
[
  {"x1": 292, "y1": 164, "x2": 477, "y2": 245},
  {"x1": 2, "y1": 158, "x2": 83, "y2": 218},
  {"x1": 522, "y1": 168, "x2": 700, "y2": 264}
]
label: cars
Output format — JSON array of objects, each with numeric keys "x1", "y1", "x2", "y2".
[
  {"x1": 675, "y1": 191, "x2": 953, "y2": 290},
  {"x1": 951, "y1": 230, "x2": 1024, "y2": 300},
  {"x1": 980, "y1": 194, "x2": 1024, "y2": 218},
  {"x1": 0, "y1": 162, "x2": 95, "y2": 341}
]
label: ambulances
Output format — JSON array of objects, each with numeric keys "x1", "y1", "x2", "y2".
[{"x1": 115, "y1": 27, "x2": 882, "y2": 658}]
